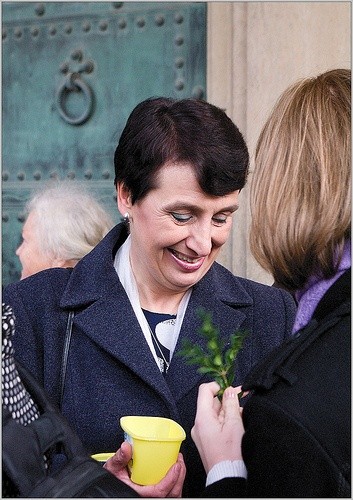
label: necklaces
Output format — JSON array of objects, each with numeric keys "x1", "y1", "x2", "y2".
[{"x1": 142, "y1": 308, "x2": 171, "y2": 374}]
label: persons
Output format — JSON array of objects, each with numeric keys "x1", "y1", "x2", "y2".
[
  {"x1": 190, "y1": 68, "x2": 351, "y2": 498},
  {"x1": 15, "y1": 187, "x2": 117, "y2": 280},
  {"x1": 2, "y1": 96, "x2": 298, "y2": 498}
]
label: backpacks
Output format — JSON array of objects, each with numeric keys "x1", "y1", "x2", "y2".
[{"x1": 1, "y1": 358, "x2": 141, "y2": 498}]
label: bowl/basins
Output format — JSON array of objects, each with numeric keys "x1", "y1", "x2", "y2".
[{"x1": 120, "y1": 416, "x2": 186, "y2": 485}]
